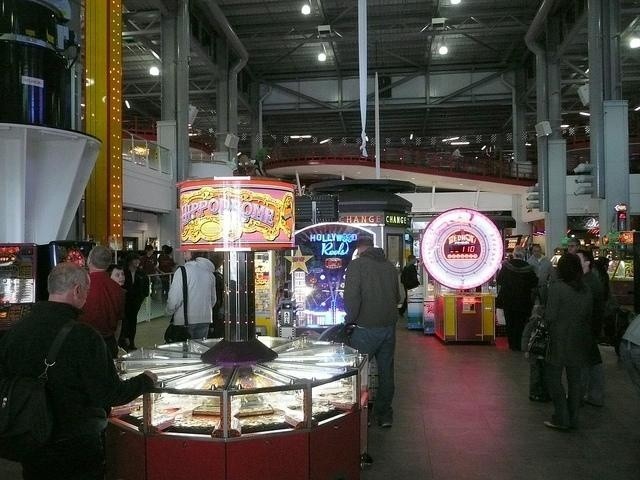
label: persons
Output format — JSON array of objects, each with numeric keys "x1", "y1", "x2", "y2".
[
  {"x1": 342, "y1": 232, "x2": 400, "y2": 429},
  {"x1": 0, "y1": 261, "x2": 160, "y2": 479},
  {"x1": 542, "y1": 252, "x2": 597, "y2": 433},
  {"x1": 496, "y1": 238, "x2": 612, "y2": 404},
  {"x1": 85, "y1": 243, "x2": 224, "y2": 355},
  {"x1": 398, "y1": 255, "x2": 422, "y2": 317},
  {"x1": 619, "y1": 315, "x2": 640, "y2": 396}
]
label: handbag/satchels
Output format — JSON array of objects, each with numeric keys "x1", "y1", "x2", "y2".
[
  {"x1": 0, "y1": 376, "x2": 52, "y2": 462},
  {"x1": 528, "y1": 327, "x2": 551, "y2": 359},
  {"x1": 164, "y1": 324, "x2": 190, "y2": 343}
]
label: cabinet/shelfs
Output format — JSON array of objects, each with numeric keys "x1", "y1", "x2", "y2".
[{"x1": 99, "y1": 335, "x2": 373, "y2": 480}]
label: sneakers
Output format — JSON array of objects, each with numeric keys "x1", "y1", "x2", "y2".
[{"x1": 374, "y1": 416, "x2": 393, "y2": 426}]
